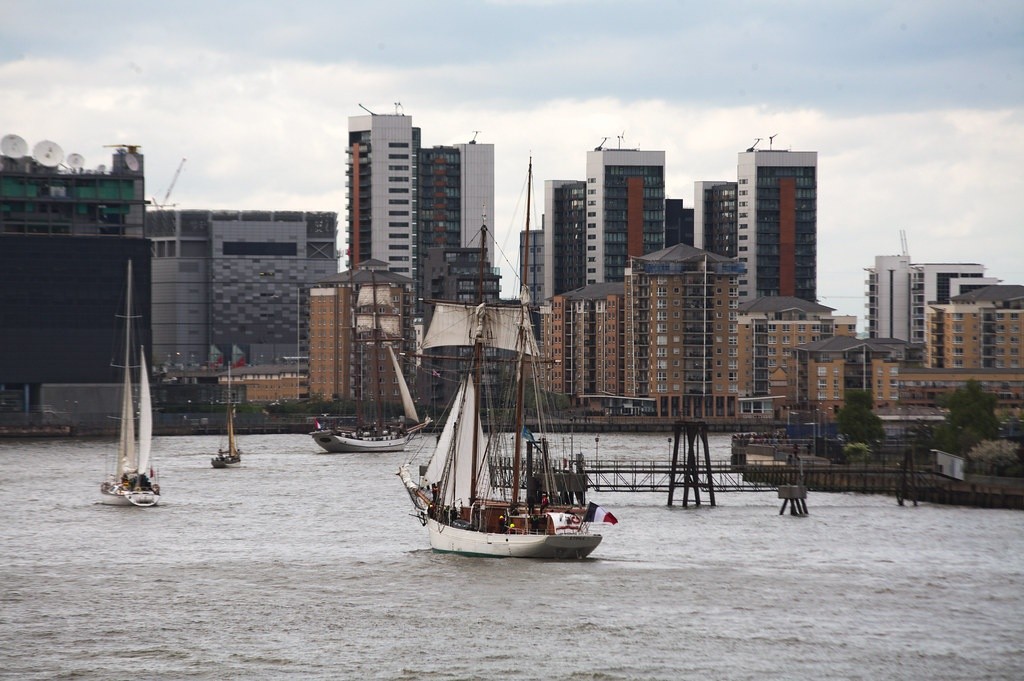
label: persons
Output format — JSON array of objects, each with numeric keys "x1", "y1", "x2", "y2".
[
  {"x1": 121, "y1": 473, "x2": 129, "y2": 490},
  {"x1": 218, "y1": 449, "x2": 224, "y2": 461},
  {"x1": 498, "y1": 515, "x2": 504, "y2": 533},
  {"x1": 793, "y1": 443, "x2": 798, "y2": 457},
  {"x1": 732, "y1": 433, "x2": 783, "y2": 447},
  {"x1": 507, "y1": 523, "x2": 516, "y2": 534},
  {"x1": 807, "y1": 443, "x2": 812, "y2": 456}
]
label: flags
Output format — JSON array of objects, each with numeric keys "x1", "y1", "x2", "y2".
[
  {"x1": 582, "y1": 502, "x2": 598, "y2": 523},
  {"x1": 522, "y1": 426, "x2": 534, "y2": 440},
  {"x1": 603, "y1": 512, "x2": 618, "y2": 525}
]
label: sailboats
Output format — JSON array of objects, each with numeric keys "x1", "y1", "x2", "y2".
[
  {"x1": 307, "y1": 268, "x2": 435, "y2": 453},
  {"x1": 394, "y1": 150, "x2": 604, "y2": 559},
  {"x1": 209, "y1": 359, "x2": 244, "y2": 469},
  {"x1": 98, "y1": 259, "x2": 162, "y2": 507}
]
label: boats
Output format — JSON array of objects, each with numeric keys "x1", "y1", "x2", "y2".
[{"x1": 107, "y1": 352, "x2": 269, "y2": 434}]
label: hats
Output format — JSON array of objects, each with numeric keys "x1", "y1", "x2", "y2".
[
  {"x1": 542, "y1": 494, "x2": 546, "y2": 496},
  {"x1": 499, "y1": 516, "x2": 504, "y2": 519}
]
